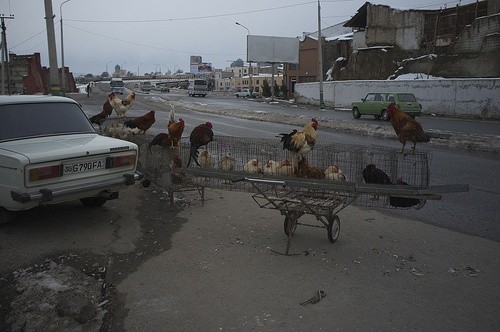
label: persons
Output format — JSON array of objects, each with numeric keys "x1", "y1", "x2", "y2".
[{"x1": 86, "y1": 83, "x2": 92, "y2": 97}]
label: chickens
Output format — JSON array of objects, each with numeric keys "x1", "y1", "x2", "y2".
[
  {"x1": 243, "y1": 158, "x2": 262, "y2": 177},
  {"x1": 89, "y1": 99, "x2": 113, "y2": 131},
  {"x1": 280, "y1": 159, "x2": 295, "y2": 180},
  {"x1": 108, "y1": 92, "x2": 135, "y2": 118},
  {"x1": 198, "y1": 150, "x2": 216, "y2": 169},
  {"x1": 167, "y1": 118, "x2": 185, "y2": 147},
  {"x1": 171, "y1": 154, "x2": 184, "y2": 184},
  {"x1": 218, "y1": 152, "x2": 235, "y2": 172},
  {"x1": 299, "y1": 156, "x2": 325, "y2": 179},
  {"x1": 362, "y1": 163, "x2": 392, "y2": 201},
  {"x1": 324, "y1": 165, "x2": 347, "y2": 181},
  {"x1": 262, "y1": 159, "x2": 279, "y2": 177},
  {"x1": 123, "y1": 110, "x2": 156, "y2": 136},
  {"x1": 386, "y1": 101, "x2": 432, "y2": 154},
  {"x1": 389, "y1": 178, "x2": 421, "y2": 207},
  {"x1": 187, "y1": 122, "x2": 214, "y2": 169},
  {"x1": 275, "y1": 117, "x2": 319, "y2": 159}
]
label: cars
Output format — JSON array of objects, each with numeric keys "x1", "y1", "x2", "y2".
[
  {"x1": 351, "y1": 91, "x2": 422, "y2": 121},
  {"x1": 235, "y1": 89, "x2": 259, "y2": 98},
  {"x1": 0, "y1": 95, "x2": 140, "y2": 225}
]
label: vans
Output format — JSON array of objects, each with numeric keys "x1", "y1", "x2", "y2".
[{"x1": 140, "y1": 80, "x2": 152, "y2": 91}]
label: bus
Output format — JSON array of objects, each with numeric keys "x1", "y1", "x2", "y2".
[{"x1": 187, "y1": 79, "x2": 208, "y2": 98}]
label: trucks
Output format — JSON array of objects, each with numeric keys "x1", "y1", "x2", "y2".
[{"x1": 110, "y1": 78, "x2": 125, "y2": 94}]
